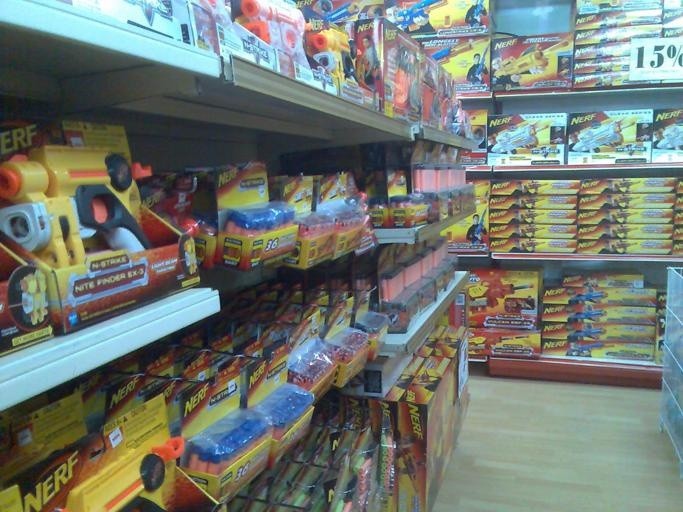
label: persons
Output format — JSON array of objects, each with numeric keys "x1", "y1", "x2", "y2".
[
  {"x1": 652, "y1": 124, "x2": 683, "y2": 150},
  {"x1": 466, "y1": 214, "x2": 488, "y2": 246},
  {"x1": 486, "y1": 128, "x2": 517, "y2": 155},
  {"x1": 466, "y1": 54, "x2": 489, "y2": 86},
  {"x1": 385, "y1": 1, "x2": 435, "y2": 35},
  {"x1": 569, "y1": 128, "x2": 600, "y2": 153},
  {"x1": 464, "y1": 1, "x2": 487, "y2": 27}
]
